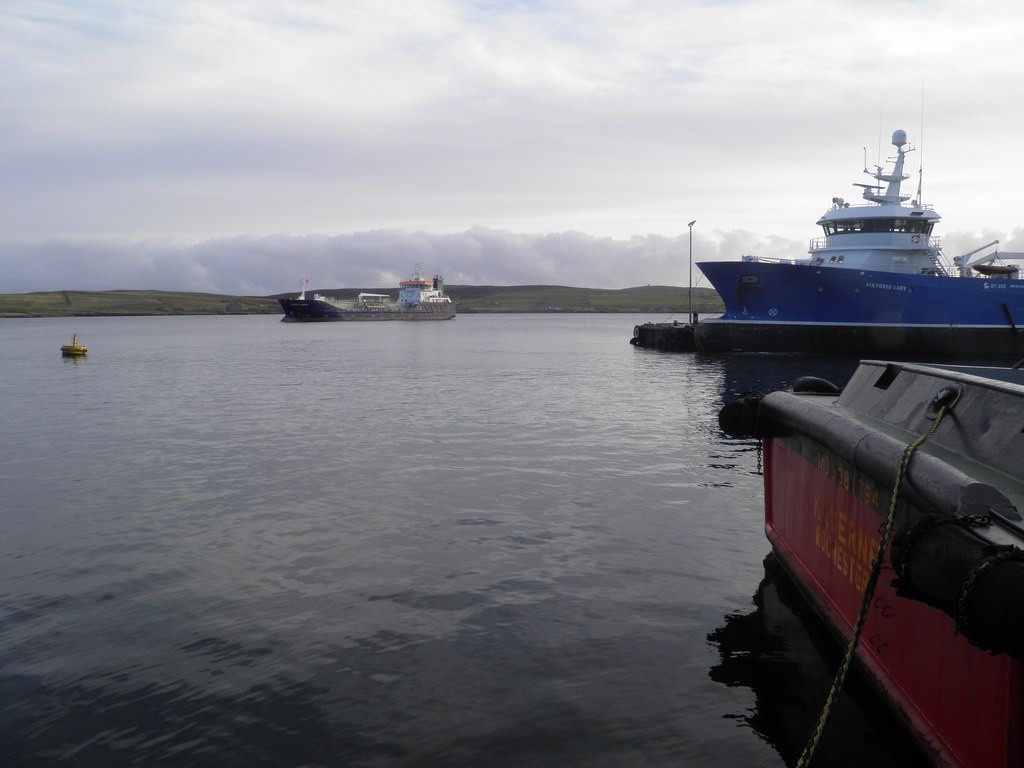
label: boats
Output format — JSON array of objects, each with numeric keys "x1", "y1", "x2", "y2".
[
  {"x1": 60, "y1": 333, "x2": 88, "y2": 355},
  {"x1": 279, "y1": 263, "x2": 455, "y2": 320},
  {"x1": 763, "y1": 359, "x2": 1024, "y2": 767},
  {"x1": 694, "y1": 130, "x2": 1024, "y2": 353}
]
